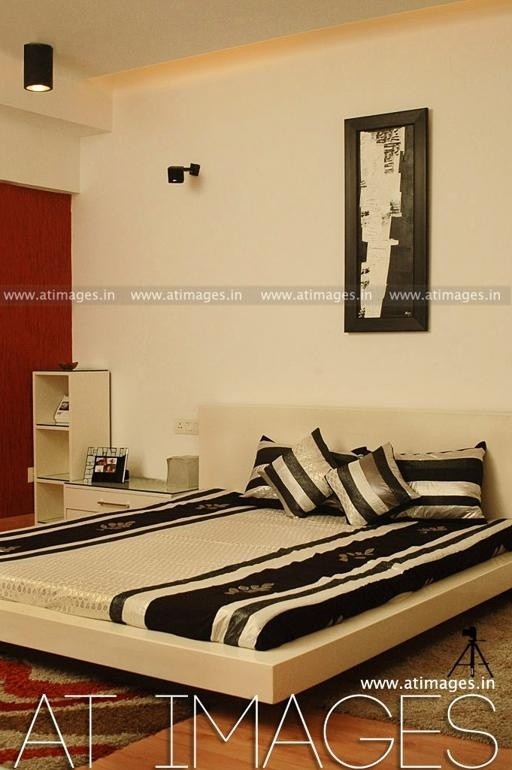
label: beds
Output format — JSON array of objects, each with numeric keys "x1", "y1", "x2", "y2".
[{"x1": 0, "y1": 402, "x2": 511, "y2": 711}]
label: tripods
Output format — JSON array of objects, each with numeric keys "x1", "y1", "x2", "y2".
[{"x1": 447, "y1": 625, "x2": 493, "y2": 678}]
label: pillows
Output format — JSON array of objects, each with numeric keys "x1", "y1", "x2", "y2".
[
  {"x1": 360, "y1": 438, "x2": 490, "y2": 528},
  {"x1": 255, "y1": 424, "x2": 343, "y2": 520},
  {"x1": 238, "y1": 430, "x2": 368, "y2": 519},
  {"x1": 322, "y1": 440, "x2": 424, "y2": 530}
]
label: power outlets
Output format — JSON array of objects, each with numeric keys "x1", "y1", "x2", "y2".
[{"x1": 173, "y1": 416, "x2": 185, "y2": 435}]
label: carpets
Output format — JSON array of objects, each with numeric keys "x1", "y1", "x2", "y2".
[
  {"x1": 270, "y1": 593, "x2": 512, "y2": 750},
  {"x1": 0, "y1": 641, "x2": 214, "y2": 769}
]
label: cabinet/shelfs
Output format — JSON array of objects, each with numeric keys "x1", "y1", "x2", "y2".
[
  {"x1": 28, "y1": 367, "x2": 115, "y2": 528},
  {"x1": 60, "y1": 475, "x2": 200, "y2": 525}
]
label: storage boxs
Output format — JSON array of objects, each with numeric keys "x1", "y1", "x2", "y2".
[{"x1": 164, "y1": 455, "x2": 200, "y2": 489}]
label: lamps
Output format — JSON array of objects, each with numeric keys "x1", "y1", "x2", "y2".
[
  {"x1": 165, "y1": 162, "x2": 200, "y2": 185},
  {"x1": 22, "y1": 40, "x2": 56, "y2": 93}
]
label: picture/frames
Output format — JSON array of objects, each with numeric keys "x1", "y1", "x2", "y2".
[
  {"x1": 338, "y1": 105, "x2": 431, "y2": 336},
  {"x1": 82, "y1": 446, "x2": 129, "y2": 484}
]
